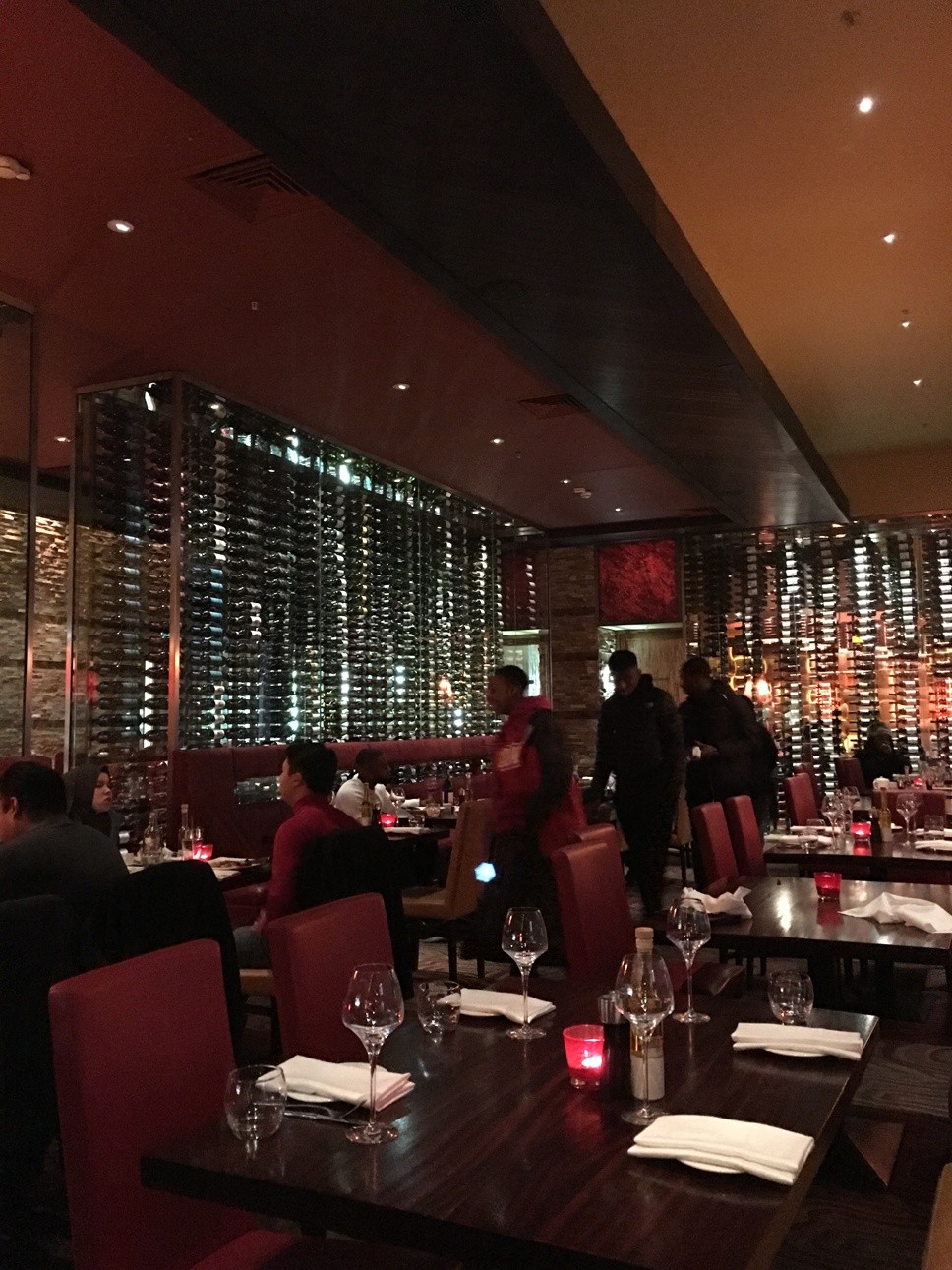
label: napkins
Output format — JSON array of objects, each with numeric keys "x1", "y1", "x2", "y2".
[
  {"x1": 791, "y1": 826, "x2": 844, "y2": 836},
  {"x1": 731, "y1": 1023, "x2": 864, "y2": 1060},
  {"x1": 255, "y1": 1054, "x2": 418, "y2": 1110},
  {"x1": 913, "y1": 828, "x2": 952, "y2": 838},
  {"x1": 913, "y1": 840, "x2": 952, "y2": 853},
  {"x1": 765, "y1": 834, "x2": 834, "y2": 848},
  {"x1": 838, "y1": 892, "x2": 952, "y2": 934},
  {"x1": 627, "y1": 1114, "x2": 816, "y2": 1185},
  {"x1": 436, "y1": 988, "x2": 555, "y2": 1023},
  {"x1": 680, "y1": 888, "x2": 753, "y2": 918}
]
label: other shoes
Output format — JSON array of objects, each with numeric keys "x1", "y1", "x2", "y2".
[{"x1": 643, "y1": 904, "x2": 661, "y2": 916}]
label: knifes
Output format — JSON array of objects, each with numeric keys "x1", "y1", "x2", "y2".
[{"x1": 285, "y1": 1110, "x2": 372, "y2": 1126}]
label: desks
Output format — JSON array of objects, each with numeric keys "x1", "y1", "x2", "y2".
[
  {"x1": 386, "y1": 827, "x2": 454, "y2": 888},
  {"x1": 139, "y1": 977, "x2": 879, "y2": 1270},
  {"x1": 874, "y1": 786, "x2": 952, "y2": 829},
  {"x1": 763, "y1": 829, "x2": 952, "y2": 885},
  {"x1": 635, "y1": 876, "x2": 952, "y2": 1022},
  {"x1": 122, "y1": 856, "x2": 273, "y2": 893},
  {"x1": 390, "y1": 785, "x2": 593, "y2": 829}
]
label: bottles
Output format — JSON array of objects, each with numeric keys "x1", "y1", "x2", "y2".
[
  {"x1": 630, "y1": 926, "x2": 666, "y2": 1100},
  {"x1": 141, "y1": 810, "x2": 165, "y2": 862},
  {"x1": 462, "y1": 772, "x2": 474, "y2": 804},
  {"x1": 880, "y1": 803, "x2": 893, "y2": 842},
  {"x1": 178, "y1": 804, "x2": 202, "y2": 861},
  {"x1": 600, "y1": 988, "x2": 631, "y2": 1098},
  {"x1": 441, "y1": 768, "x2": 455, "y2": 805}
]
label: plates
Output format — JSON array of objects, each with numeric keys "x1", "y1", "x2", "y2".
[
  {"x1": 766, "y1": 1048, "x2": 826, "y2": 1058},
  {"x1": 206, "y1": 856, "x2": 266, "y2": 869},
  {"x1": 891, "y1": 826, "x2": 903, "y2": 829},
  {"x1": 284, "y1": 1062, "x2": 387, "y2": 1103},
  {"x1": 460, "y1": 1009, "x2": 501, "y2": 1018},
  {"x1": 675, "y1": 1158, "x2": 745, "y2": 1173}
]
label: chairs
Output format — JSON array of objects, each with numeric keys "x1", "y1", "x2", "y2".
[{"x1": 0, "y1": 757, "x2": 948, "y2": 1270}]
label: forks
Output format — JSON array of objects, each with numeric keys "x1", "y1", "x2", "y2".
[{"x1": 251, "y1": 1098, "x2": 368, "y2": 1118}]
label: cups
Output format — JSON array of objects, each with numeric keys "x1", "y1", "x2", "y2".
[
  {"x1": 797, "y1": 829, "x2": 819, "y2": 849},
  {"x1": 222, "y1": 1064, "x2": 288, "y2": 1144},
  {"x1": 426, "y1": 803, "x2": 441, "y2": 817},
  {"x1": 193, "y1": 845, "x2": 213, "y2": 861},
  {"x1": 806, "y1": 819, "x2": 826, "y2": 836},
  {"x1": 814, "y1": 871, "x2": 842, "y2": 901},
  {"x1": 924, "y1": 814, "x2": 945, "y2": 840},
  {"x1": 561, "y1": 1025, "x2": 605, "y2": 1090},
  {"x1": 415, "y1": 979, "x2": 464, "y2": 1035},
  {"x1": 766, "y1": 968, "x2": 816, "y2": 1026},
  {"x1": 407, "y1": 810, "x2": 426, "y2": 828},
  {"x1": 850, "y1": 822, "x2": 871, "y2": 842}
]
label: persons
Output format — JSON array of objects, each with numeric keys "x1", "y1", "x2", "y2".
[
  {"x1": 333, "y1": 749, "x2": 415, "y2": 828},
  {"x1": 231, "y1": 741, "x2": 366, "y2": 971},
  {"x1": 0, "y1": 759, "x2": 148, "y2": 1205},
  {"x1": 478, "y1": 665, "x2": 588, "y2": 971},
  {"x1": 587, "y1": 649, "x2": 782, "y2": 926},
  {"x1": 852, "y1": 722, "x2": 911, "y2": 798}
]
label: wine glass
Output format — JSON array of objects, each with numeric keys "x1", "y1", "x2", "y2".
[
  {"x1": 340, "y1": 761, "x2": 952, "y2": 1144},
  {"x1": 359, "y1": 781, "x2": 372, "y2": 827}
]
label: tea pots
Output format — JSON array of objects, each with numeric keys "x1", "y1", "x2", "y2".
[{"x1": 873, "y1": 776, "x2": 890, "y2": 790}]
